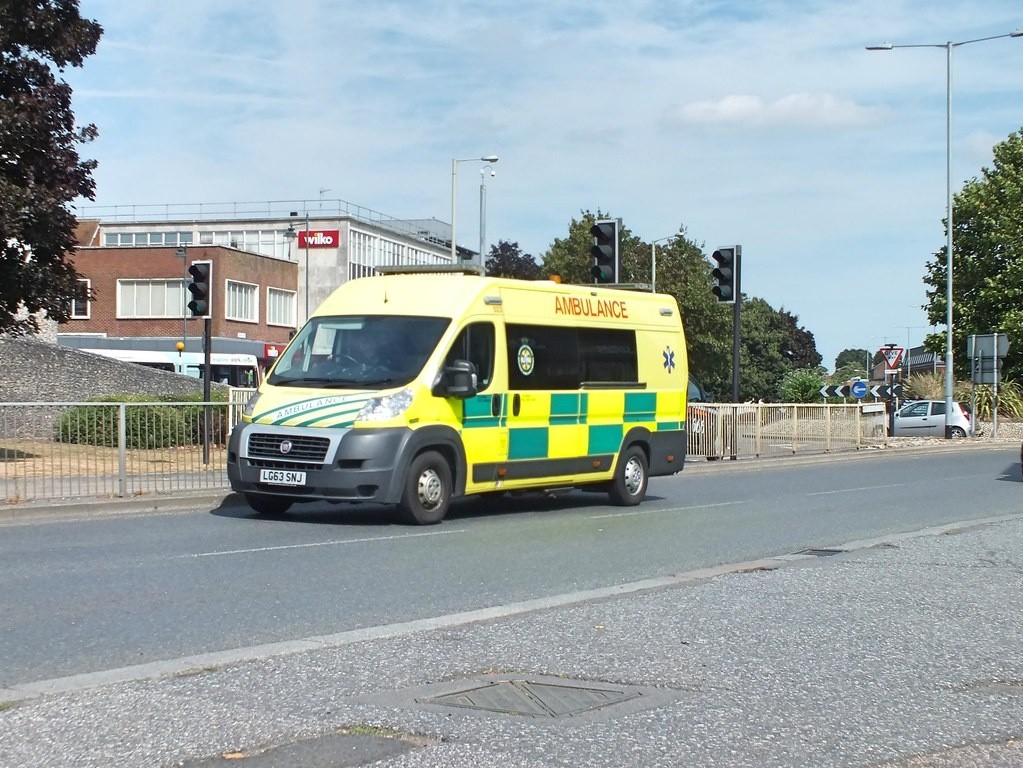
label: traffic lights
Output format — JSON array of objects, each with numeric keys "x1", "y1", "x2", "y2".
[
  {"x1": 712, "y1": 246, "x2": 736, "y2": 304},
  {"x1": 188, "y1": 259, "x2": 213, "y2": 319},
  {"x1": 590, "y1": 219, "x2": 619, "y2": 285}
]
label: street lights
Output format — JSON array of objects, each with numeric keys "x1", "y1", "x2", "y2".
[
  {"x1": 451, "y1": 155, "x2": 499, "y2": 265},
  {"x1": 864, "y1": 29, "x2": 1023, "y2": 442},
  {"x1": 283, "y1": 213, "x2": 309, "y2": 321},
  {"x1": 480, "y1": 165, "x2": 495, "y2": 276},
  {"x1": 652, "y1": 231, "x2": 686, "y2": 293}
]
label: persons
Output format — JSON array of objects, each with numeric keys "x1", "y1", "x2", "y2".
[{"x1": 345, "y1": 335, "x2": 403, "y2": 373}]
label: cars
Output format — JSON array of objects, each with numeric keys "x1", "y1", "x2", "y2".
[
  {"x1": 686, "y1": 372, "x2": 725, "y2": 460},
  {"x1": 864, "y1": 400, "x2": 983, "y2": 437}
]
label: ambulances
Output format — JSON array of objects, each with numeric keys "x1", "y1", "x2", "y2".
[{"x1": 227, "y1": 265, "x2": 689, "y2": 525}]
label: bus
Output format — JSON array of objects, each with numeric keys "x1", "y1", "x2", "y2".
[{"x1": 80, "y1": 348, "x2": 260, "y2": 393}]
